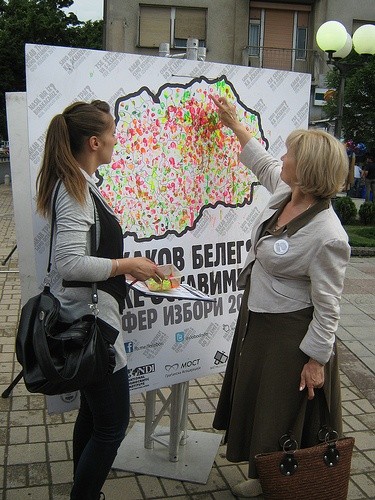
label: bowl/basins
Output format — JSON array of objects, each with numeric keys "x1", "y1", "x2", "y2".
[{"x1": 144, "y1": 264, "x2": 183, "y2": 291}]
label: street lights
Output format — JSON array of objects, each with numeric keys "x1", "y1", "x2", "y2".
[{"x1": 317, "y1": 18, "x2": 375, "y2": 141}]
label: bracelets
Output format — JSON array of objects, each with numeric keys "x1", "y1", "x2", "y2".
[{"x1": 113, "y1": 259, "x2": 119, "y2": 278}]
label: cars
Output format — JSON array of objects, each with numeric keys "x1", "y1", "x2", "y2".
[{"x1": 345, "y1": 140, "x2": 369, "y2": 163}]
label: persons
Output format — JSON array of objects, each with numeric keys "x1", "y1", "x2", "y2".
[
  {"x1": 36, "y1": 100, "x2": 164, "y2": 500},
  {"x1": 353, "y1": 165, "x2": 363, "y2": 192},
  {"x1": 210, "y1": 94, "x2": 351, "y2": 500}
]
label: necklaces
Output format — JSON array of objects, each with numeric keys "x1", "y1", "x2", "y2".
[{"x1": 277, "y1": 203, "x2": 311, "y2": 221}]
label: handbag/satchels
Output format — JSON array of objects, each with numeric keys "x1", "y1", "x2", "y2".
[
  {"x1": 254, "y1": 437, "x2": 354, "y2": 500},
  {"x1": 14, "y1": 293, "x2": 119, "y2": 393}
]
